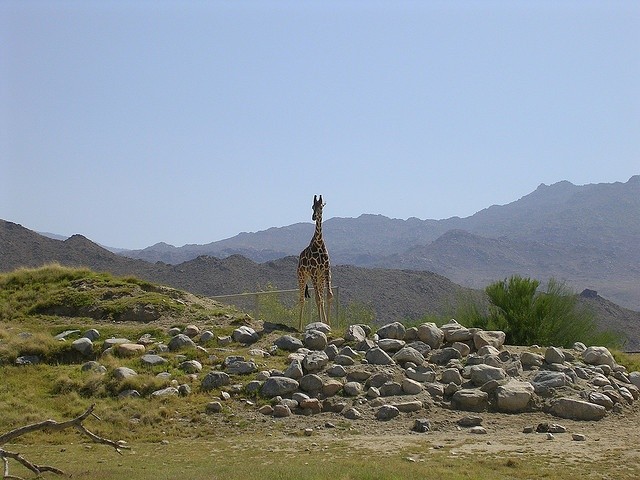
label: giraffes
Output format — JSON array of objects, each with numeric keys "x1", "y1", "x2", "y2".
[{"x1": 296, "y1": 194, "x2": 334, "y2": 333}]
